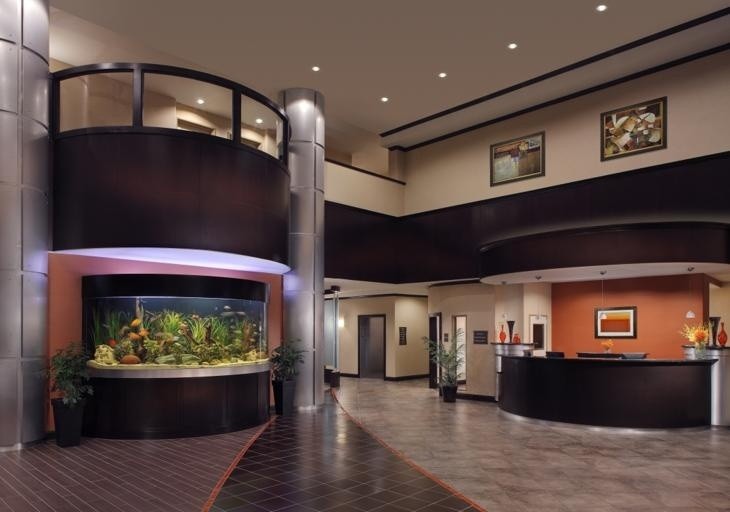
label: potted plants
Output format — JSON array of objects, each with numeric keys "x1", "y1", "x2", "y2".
[
  {"x1": 268, "y1": 337, "x2": 310, "y2": 416},
  {"x1": 40, "y1": 343, "x2": 94, "y2": 448},
  {"x1": 421, "y1": 328, "x2": 467, "y2": 402}
]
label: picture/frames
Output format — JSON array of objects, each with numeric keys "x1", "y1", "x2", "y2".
[
  {"x1": 490, "y1": 130, "x2": 545, "y2": 187},
  {"x1": 600, "y1": 96, "x2": 667, "y2": 162},
  {"x1": 594, "y1": 306, "x2": 637, "y2": 339}
]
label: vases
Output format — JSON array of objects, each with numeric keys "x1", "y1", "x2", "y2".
[{"x1": 696, "y1": 343, "x2": 707, "y2": 360}]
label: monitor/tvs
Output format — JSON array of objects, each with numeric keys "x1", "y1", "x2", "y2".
[
  {"x1": 621, "y1": 353, "x2": 647, "y2": 360},
  {"x1": 546, "y1": 351, "x2": 564, "y2": 358}
]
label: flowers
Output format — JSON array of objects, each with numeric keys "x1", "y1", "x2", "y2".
[{"x1": 676, "y1": 320, "x2": 714, "y2": 353}]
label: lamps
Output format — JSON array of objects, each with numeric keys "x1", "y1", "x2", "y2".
[
  {"x1": 535, "y1": 276, "x2": 542, "y2": 320},
  {"x1": 599, "y1": 271, "x2": 607, "y2": 319},
  {"x1": 686, "y1": 266, "x2": 695, "y2": 319},
  {"x1": 502, "y1": 281, "x2": 508, "y2": 318}
]
